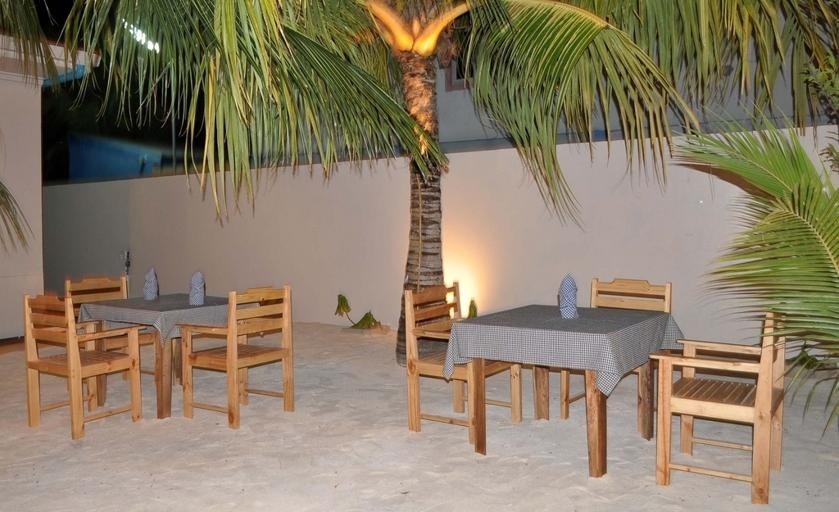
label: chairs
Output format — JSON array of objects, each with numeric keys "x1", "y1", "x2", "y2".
[
  {"x1": 559, "y1": 280, "x2": 676, "y2": 439},
  {"x1": 405, "y1": 281, "x2": 526, "y2": 445},
  {"x1": 22, "y1": 290, "x2": 149, "y2": 439},
  {"x1": 64, "y1": 275, "x2": 158, "y2": 405},
  {"x1": 174, "y1": 282, "x2": 293, "y2": 431},
  {"x1": 645, "y1": 301, "x2": 787, "y2": 505}
]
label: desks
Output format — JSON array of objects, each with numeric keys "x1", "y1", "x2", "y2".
[
  {"x1": 440, "y1": 303, "x2": 685, "y2": 479},
  {"x1": 76, "y1": 293, "x2": 259, "y2": 420}
]
